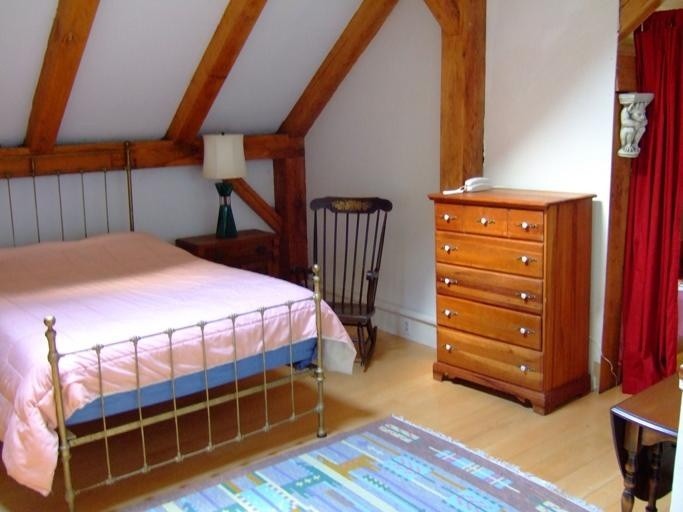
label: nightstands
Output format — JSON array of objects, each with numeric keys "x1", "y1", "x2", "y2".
[{"x1": 174, "y1": 228, "x2": 281, "y2": 277}]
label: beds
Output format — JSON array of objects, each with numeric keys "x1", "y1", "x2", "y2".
[{"x1": 1, "y1": 139, "x2": 357, "y2": 512}]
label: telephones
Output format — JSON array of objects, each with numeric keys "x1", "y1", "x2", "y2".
[{"x1": 464, "y1": 176, "x2": 491, "y2": 192}]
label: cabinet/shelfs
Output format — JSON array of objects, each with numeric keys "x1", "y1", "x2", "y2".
[{"x1": 427, "y1": 188, "x2": 596, "y2": 416}]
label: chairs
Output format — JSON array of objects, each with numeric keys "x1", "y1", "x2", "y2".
[{"x1": 289, "y1": 196, "x2": 392, "y2": 370}]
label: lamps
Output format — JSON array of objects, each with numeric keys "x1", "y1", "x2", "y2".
[{"x1": 202, "y1": 131, "x2": 247, "y2": 239}]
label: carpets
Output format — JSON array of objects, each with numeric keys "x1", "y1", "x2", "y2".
[{"x1": 124, "y1": 412, "x2": 603, "y2": 512}]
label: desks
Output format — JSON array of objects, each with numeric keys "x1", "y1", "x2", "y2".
[{"x1": 610, "y1": 369, "x2": 683, "y2": 512}]
